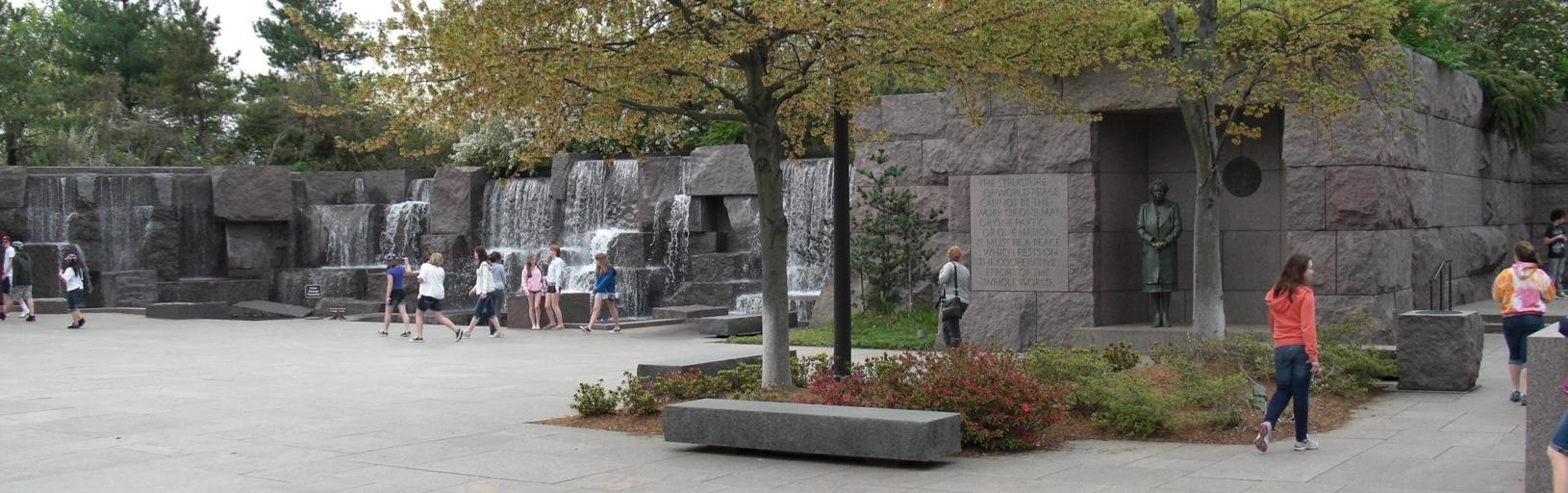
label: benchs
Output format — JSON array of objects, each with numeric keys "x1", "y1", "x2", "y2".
[
  {"x1": 699, "y1": 310, "x2": 798, "y2": 338},
  {"x1": 636, "y1": 350, "x2": 797, "y2": 390},
  {"x1": 661, "y1": 396, "x2": 964, "y2": 470}
]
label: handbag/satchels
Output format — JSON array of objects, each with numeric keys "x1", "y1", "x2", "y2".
[
  {"x1": 609, "y1": 293, "x2": 616, "y2": 300},
  {"x1": 940, "y1": 297, "x2": 963, "y2": 322}
]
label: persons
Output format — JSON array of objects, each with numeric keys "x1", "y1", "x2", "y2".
[
  {"x1": 521, "y1": 254, "x2": 547, "y2": 331},
  {"x1": 1135, "y1": 178, "x2": 1183, "y2": 328},
  {"x1": 937, "y1": 245, "x2": 971, "y2": 348},
  {"x1": 0, "y1": 236, "x2": 36, "y2": 322},
  {"x1": 543, "y1": 246, "x2": 567, "y2": 330},
  {"x1": 579, "y1": 253, "x2": 621, "y2": 334},
  {"x1": 1546, "y1": 408, "x2": 1568, "y2": 493},
  {"x1": 377, "y1": 248, "x2": 507, "y2": 341},
  {"x1": 1491, "y1": 240, "x2": 1556, "y2": 406},
  {"x1": 59, "y1": 254, "x2": 86, "y2": 328},
  {"x1": 1253, "y1": 255, "x2": 1322, "y2": 451},
  {"x1": 1543, "y1": 208, "x2": 1567, "y2": 300}
]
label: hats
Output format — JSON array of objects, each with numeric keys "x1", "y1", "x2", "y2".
[
  {"x1": 2, "y1": 236, "x2": 10, "y2": 240},
  {"x1": 64, "y1": 254, "x2": 76, "y2": 262},
  {"x1": 12, "y1": 240, "x2": 22, "y2": 248},
  {"x1": 385, "y1": 252, "x2": 397, "y2": 261}
]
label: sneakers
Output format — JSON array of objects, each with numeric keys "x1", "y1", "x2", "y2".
[
  {"x1": 532, "y1": 323, "x2": 565, "y2": 330},
  {"x1": 378, "y1": 331, "x2": 388, "y2": 336},
  {"x1": 1520, "y1": 394, "x2": 1527, "y2": 405},
  {"x1": 489, "y1": 331, "x2": 503, "y2": 338},
  {"x1": 1293, "y1": 434, "x2": 1318, "y2": 449},
  {"x1": 455, "y1": 327, "x2": 463, "y2": 341},
  {"x1": 0, "y1": 310, "x2": 36, "y2": 320},
  {"x1": 410, "y1": 336, "x2": 423, "y2": 343},
  {"x1": 1510, "y1": 390, "x2": 1520, "y2": 401},
  {"x1": 1255, "y1": 421, "x2": 1272, "y2": 453},
  {"x1": 609, "y1": 328, "x2": 620, "y2": 334},
  {"x1": 579, "y1": 326, "x2": 591, "y2": 333},
  {"x1": 68, "y1": 322, "x2": 79, "y2": 327},
  {"x1": 79, "y1": 318, "x2": 85, "y2": 326},
  {"x1": 400, "y1": 331, "x2": 411, "y2": 337},
  {"x1": 461, "y1": 330, "x2": 470, "y2": 338}
]
